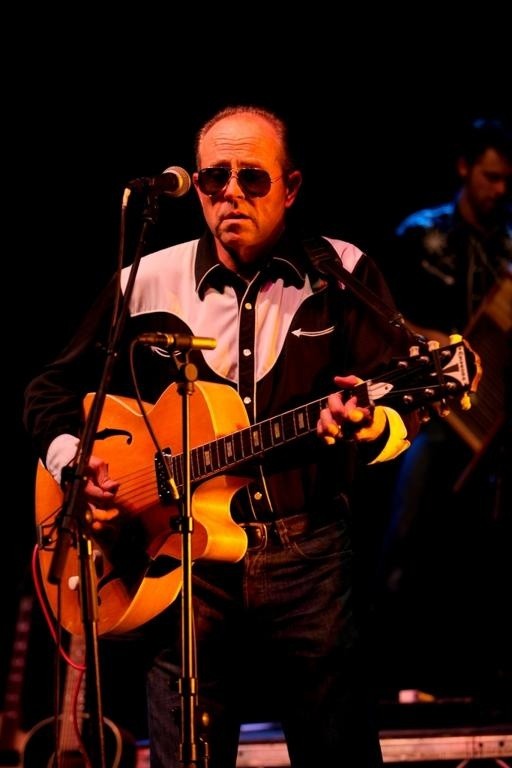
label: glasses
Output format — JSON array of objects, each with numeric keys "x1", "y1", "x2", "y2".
[{"x1": 195, "y1": 166, "x2": 280, "y2": 196}]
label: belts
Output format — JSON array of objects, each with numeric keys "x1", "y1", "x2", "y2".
[{"x1": 238, "y1": 514, "x2": 313, "y2": 555}]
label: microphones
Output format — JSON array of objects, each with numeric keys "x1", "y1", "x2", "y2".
[
  {"x1": 140, "y1": 331, "x2": 217, "y2": 350},
  {"x1": 124, "y1": 165, "x2": 192, "y2": 198}
]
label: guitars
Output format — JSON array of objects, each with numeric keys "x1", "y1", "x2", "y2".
[
  {"x1": 34, "y1": 331, "x2": 481, "y2": 637},
  {"x1": 0, "y1": 598, "x2": 31, "y2": 764},
  {"x1": 22, "y1": 636, "x2": 137, "y2": 768}
]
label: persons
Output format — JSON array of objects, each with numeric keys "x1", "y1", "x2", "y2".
[
  {"x1": 23, "y1": 106, "x2": 430, "y2": 768},
  {"x1": 393, "y1": 114, "x2": 512, "y2": 332}
]
